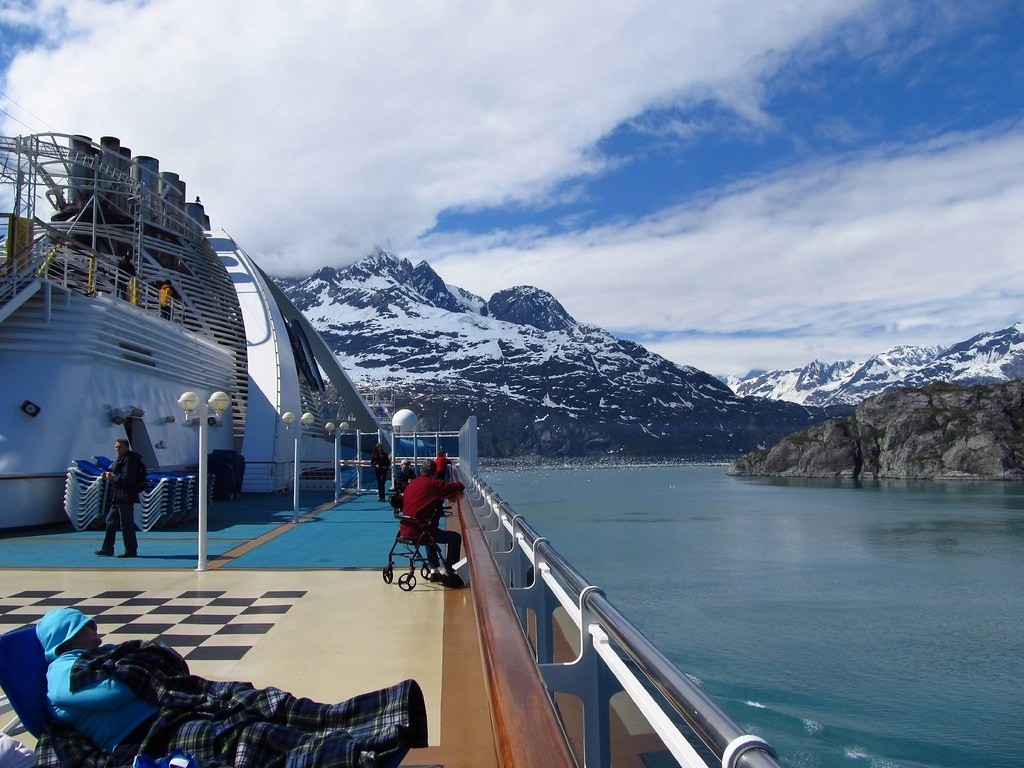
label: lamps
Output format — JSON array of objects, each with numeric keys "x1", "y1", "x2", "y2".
[
  {"x1": 184, "y1": 417, "x2": 200, "y2": 427},
  {"x1": 110, "y1": 407, "x2": 126, "y2": 426},
  {"x1": 160, "y1": 415, "x2": 176, "y2": 424},
  {"x1": 156, "y1": 440, "x2": 167, "y2": 449},
  {"x1": 128, "y1": 405, "x2": 145, "y2": 417}
]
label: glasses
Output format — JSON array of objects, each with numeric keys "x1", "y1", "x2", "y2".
[{"x1": 86, "y1": 622, "x2": 97, "y2": 632}]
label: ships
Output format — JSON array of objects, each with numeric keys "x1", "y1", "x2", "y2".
[{"x1": 2, "y1": 134, "x2": 788, "y2": 767}]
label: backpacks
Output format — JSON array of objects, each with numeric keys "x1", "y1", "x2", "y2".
[{"x1": 126, "y1": 451, "x2": 146, "y2": 493}]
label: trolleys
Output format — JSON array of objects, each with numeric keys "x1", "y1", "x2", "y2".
[{"x1": 383, "y1": 495, "x2": 457, "y2": 591}]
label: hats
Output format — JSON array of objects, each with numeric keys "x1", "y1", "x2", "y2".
[{"x1": 400, "y1": 459, "x2": 411, "y2": 466}]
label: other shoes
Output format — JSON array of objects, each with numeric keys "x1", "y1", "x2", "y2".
[
  {"x1": 95, "y1": 549, "x2": 114, "y2": 556},
  {"x1": 442, "y1": 573, "x2": 464, "y2": 587},
  {"x1": 430, "y1": 570, "x2": 444, "y2": 582},
  {"x1": 117, "y1": 550, "x2": 137, "y2": 557}
]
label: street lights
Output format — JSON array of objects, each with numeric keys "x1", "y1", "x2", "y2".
[
  {"x1": 179, "y1": 391, "x2": 230, "y2": 571},
  {"x1": 283, "y1": 412, "x2": 314, "y2": 522},
  {"x1": 326, "y1": 422, "x2": 349, "y2": 502}
]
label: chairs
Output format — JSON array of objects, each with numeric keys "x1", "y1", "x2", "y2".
[
  {"x1": 0, "y1": 627, "x2": 429, "y2": 768},
  {"x1": 63, "y1": 456, "x2": 215, "y2": 532}
]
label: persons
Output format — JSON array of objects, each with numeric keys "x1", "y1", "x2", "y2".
[
  {"x1": 436, "y1": 451, "x2": 451, "y2": 480},
  {"x1": 401, "y1": 462, "x2": 465, "y2": 585},
  {"x1": 36, "y1": 608, "x2": 428, "y2": 768},
  {"x1": 117, "y1": 255, "x2": 136, "y2": 300},
  {"x1": 94, "y1": 439, "x2": 139, "y2": 558},
  {"x1": 160, "y1": 279, "x2": 172, "y2": 321},
  {"x1": 393, "y1": 459, "x2": 416, "y2": 515},
  {"x1": 371, "y1": 443, "x2": 391, "y2": 502}
]
